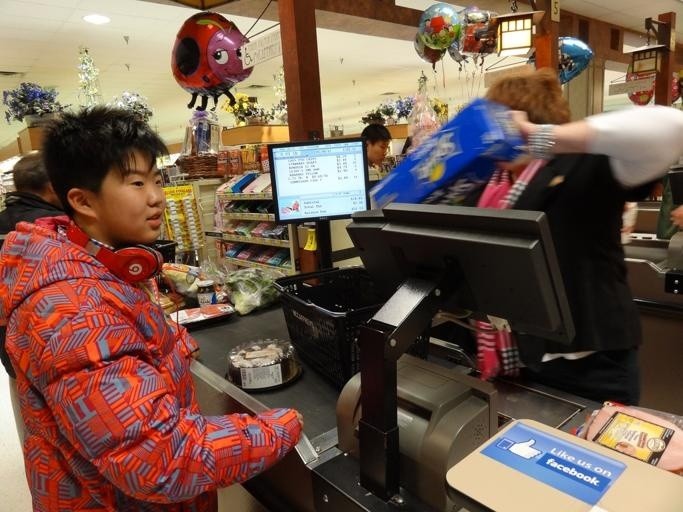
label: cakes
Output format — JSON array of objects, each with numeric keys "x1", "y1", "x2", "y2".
[{"x1": 228, "y1": 338, "x2": 299, "y2": 390}]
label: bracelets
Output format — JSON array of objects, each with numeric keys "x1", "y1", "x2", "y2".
[{"x1": 527, "y1": 122, "x2": 558, "y2": 159}]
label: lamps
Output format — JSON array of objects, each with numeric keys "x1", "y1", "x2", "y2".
[
  {"x1": 491, "y1": 0, "x2": 547, "y2": 58},
  {"x1": 626, "y1": 30, "x2": 668, "y2": 76}
]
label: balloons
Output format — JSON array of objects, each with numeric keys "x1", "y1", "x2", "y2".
[
  {"x1": 413, "y1": 3, "x2": 501, "y2": 71},
  {"x1": 170, "y1": 12, "x2": 254, "y2": 112},
  {"x1": 526, "y1": 37, "x2": 593, "y2": 85}
]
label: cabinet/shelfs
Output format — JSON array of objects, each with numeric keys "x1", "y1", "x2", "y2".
[{"x1": 220, "y1": 192, "x2": 301, "y2": 277}]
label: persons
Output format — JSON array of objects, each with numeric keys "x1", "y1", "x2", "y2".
[
  {"x1": 0, "y1": 151, "x2": 65, "y2": 454},
  {"x1": 459, "y1": 65, "x2": 683, "y2": 405},
  {"x1": 0, "y1": 103, "x2": 305, "y2": 512},
  {"x1": 360, "y1": 123, "x2": 393, "y2": 170}
]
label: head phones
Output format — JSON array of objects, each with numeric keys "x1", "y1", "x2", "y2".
[{"x1": 67, "y1": 220, "x2": 163, "y2": 285}]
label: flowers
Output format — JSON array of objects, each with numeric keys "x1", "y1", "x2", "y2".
[
  {"x1": 2, "y1": 45, "x2": 153, "y2": 128},
  {"x1": 219, "y1": 66, "x2": 289, "y2": 127},
  {"x1": 359, "y1": 95, "x2": 448, "y2": 125}
]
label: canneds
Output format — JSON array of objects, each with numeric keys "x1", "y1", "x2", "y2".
[{"x1": 195, "y1": 279, "x2": 217, "y2": 308}]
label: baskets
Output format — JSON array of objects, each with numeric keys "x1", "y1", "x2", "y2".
[
  {"x1": 175, "y1": 153, "x2": 225, "y2": 178},
  {"x1": 276, "y1": 265, "x2": 433, "y2": 393},
  {"x1": 154, "y1": 240, "x2": 177, "y2": 265}
]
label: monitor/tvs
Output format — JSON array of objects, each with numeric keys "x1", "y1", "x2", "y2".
[
  {"x1": 381, "y1": 201, "x2": 577, "y2": 347},
  {"x1": 267, "y1": 137, "x2": 372, "y2": 226},
  {"x1": 344, "y1": 210, "x2": 395, "y2": 284}
]
